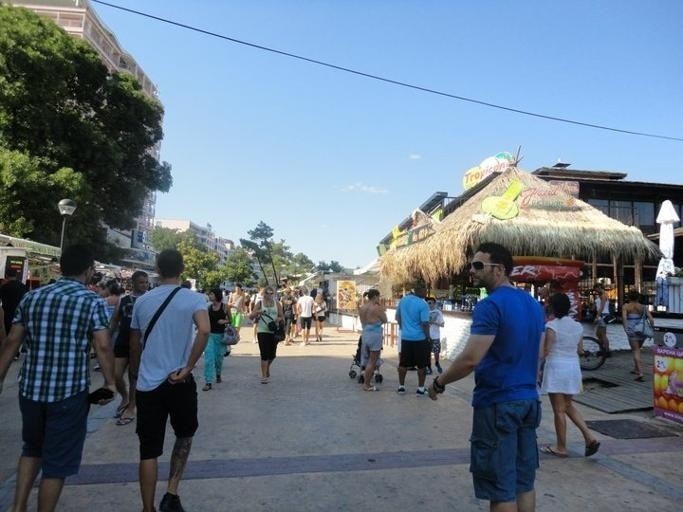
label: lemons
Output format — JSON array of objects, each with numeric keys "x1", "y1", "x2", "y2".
[{"x1": 654, "y1": 357, "x2": 683, "y2": 414}]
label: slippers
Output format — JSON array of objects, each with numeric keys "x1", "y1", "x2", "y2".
[
  {"x1": 539, "y1": 444, "x2": 568, "y2": 457},
  {"x1": 113, "y1": 402, "x2": 129, "y2": 419},
  {"x1": 364, "y1": 382, "x2": 379, "y2": 391},
  {"x1": 115, "y1": 415, "x2": 135, "y2": 424},
  {"x1": 584, "y1": 440, "x2": 601, "y2": 457}
]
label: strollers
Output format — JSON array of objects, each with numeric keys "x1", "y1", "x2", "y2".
[{"x1": 345, "y1": 331, "x2": 384, "y2": 385}]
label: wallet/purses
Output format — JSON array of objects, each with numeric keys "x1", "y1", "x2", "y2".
[{"x1": 88, "y1": 388, "x2": 113, "y2": 405}]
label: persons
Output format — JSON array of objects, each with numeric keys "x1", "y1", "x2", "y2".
[
  {"x1": 354, "y1": 292, "x2": 444, "y2": 375},
  {"x1": 0, "y1": 244, "x2": 116, "y2": 511},
  {"x1": 593, "y1": 284, "x2": 612, "y2": 356},
  {"x1": 126, "y1": 248, "x2": 214, "y2": 511},
  {"x1": 392, "y1": 286, "x2": 431, "y2": 397},
  {"x1": 538, "y1": 292, "x2": 601, "y2": 458},
  {"x1": 425, "y1": 241, "x2": 545, "y2": 511},
  {"x1": 535, "y1": 289, "x2": 547, "y2": 304},
  {"x1": 247, "y1": 285, "x2": 284, "y2": 384},
  {"x1": 194, "y1": 286, "x2": 232, "y2": 391},
  {"x1": 104, "y1": 270, "x2": 150, "y2": 425},
  {"x1": 621, "y1": 289, "x2": 654, "y2": 381},
  {"x1": 358, "y1": 289, "x2": 387, "y2": 391},
  {"x1": 577, "y1": 286, "x2": 597, "y2": 313}
]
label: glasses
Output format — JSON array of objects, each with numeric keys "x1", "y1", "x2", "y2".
[
  {"x1": 265, "y1": 292, "x2": 274, "y2": 296},
  {"x1": 468, "y1": 261, "x2": 502, "y2": 270}
]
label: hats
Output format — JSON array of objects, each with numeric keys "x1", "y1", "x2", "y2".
[{"x1": 424, "y1": 296, "x2": 437, "y2": 304}]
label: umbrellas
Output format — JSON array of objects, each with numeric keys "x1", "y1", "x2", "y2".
[{"x1": 653, "y1": 200, "x2": 681, "y2": 281}]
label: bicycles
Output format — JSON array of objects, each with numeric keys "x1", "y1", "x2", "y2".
[{"x1": 577, "y1": 335, "x2": 607, "y2": 369}]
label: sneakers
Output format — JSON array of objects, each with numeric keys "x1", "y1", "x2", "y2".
[
  {"x1": 427, "y1": 361, "x2": 443, "y2": 374},
  {"x1": 284, "y1": 333, "x2": 322, "y2": 347},
  {"x1": 203, "y1": 383, "x2": 212, "y2": 391},
  {"x1": 416, "y1": 387, "x2": 425, "y2": 396},
  {"x1": 157, "y1": 492, "x2": 185, "y2": 512},
  {"x1": 217, "y1": 375, "x2": 221, "y2": 383},
  {"x1": 629, "y1": 369, "x2": 644, "y2": 380},
  {"x1": 397, "y1": 384, "x2": 406, "y2": 394},
  {"x1": 261, "y1": 376, "x2": 269, "y2": 384}
]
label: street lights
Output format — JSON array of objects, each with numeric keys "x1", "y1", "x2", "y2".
[{"x1": 54, "y1": 197, "x2": 77, "y2": 257}]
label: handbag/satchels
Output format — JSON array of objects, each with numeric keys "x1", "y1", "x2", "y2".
[
  {"x1": 604, "y1": 313, "x2": 615, "y2": 324},
  {"x1": 221, "y1": 320, "x2": 240, "y2": 346},
  {"x1": 634, "y1": 306, "x2": 655, "y2": 338},
  {"x1": 259, "y1": 311, "x2": 278, "y2": 332}
]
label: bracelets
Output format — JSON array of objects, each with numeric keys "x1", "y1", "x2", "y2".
[{"x1": 432, "y1": 376, "x2": 444, "y2": 394}]
